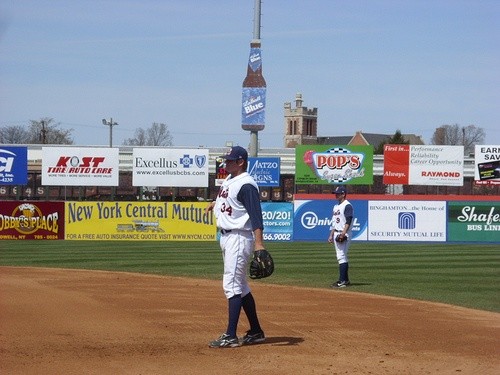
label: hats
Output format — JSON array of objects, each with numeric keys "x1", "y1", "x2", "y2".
[
  {"x1": 219, "y1": 146, "x2": 248, "y2": 161},
  {"x1": 331, "y1": 186, "x2": 347, "y2": 194}
]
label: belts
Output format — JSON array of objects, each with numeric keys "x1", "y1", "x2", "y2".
[
  {"x1": 334, "y1": 229, "x2": 342, "y2": 232},
  {"x1": 220, "y1": 229, "x2": 232, "y2": 234}
]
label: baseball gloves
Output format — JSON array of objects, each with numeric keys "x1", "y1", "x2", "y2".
[
  {"x1": 335, "y1": 234, "x2": 349, "y2": 243},
  {"x1": 248, "y1": 249, "x2": 276, "y2": 280}
]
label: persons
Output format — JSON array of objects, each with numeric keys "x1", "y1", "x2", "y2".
[
  {"x1": 328, "y1": 185, "x2": 353, "y2": 287},
  {"x1": 208, "y1": 146, "x2": 265, "y2": 348}
]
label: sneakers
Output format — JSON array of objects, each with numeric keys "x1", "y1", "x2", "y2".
[
  {"x1": 329, "y1": 280, "x2": 350, "y2": 288},
  {"x1": 206, "y1": 332, "x2": 240, "y2": 348},
  {"x1": 238, "y1": 330, "x2": 265, "y2": 345}
]
